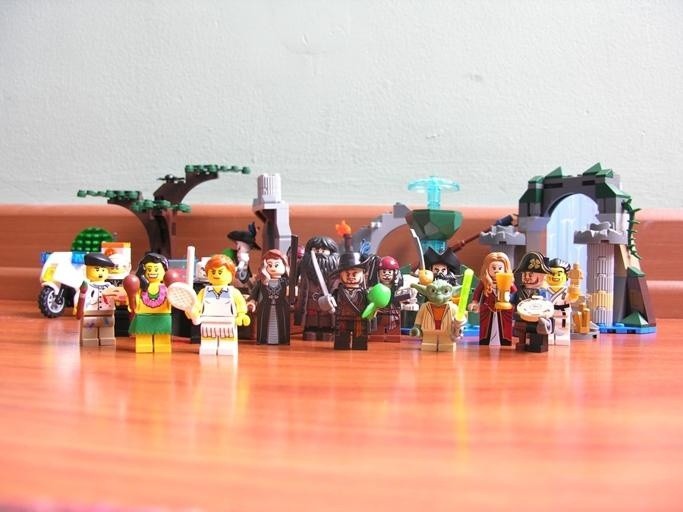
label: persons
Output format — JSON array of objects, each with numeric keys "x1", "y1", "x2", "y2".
[
  {"x1": 294, "y1": 235, "x2": 403, "y2": 349},
  {"x1": 73, "y1": 222, "x2": 292, "y2": 355},
  {"x1": 469, "y1": 250, "x2": 584, "y2": 353},
  {"x1": 415, "y1": 247, "x2": 460, "y2": 305},
  {"x1": 38, "y1": 162, "x2": 656, "y2": 355}
]
